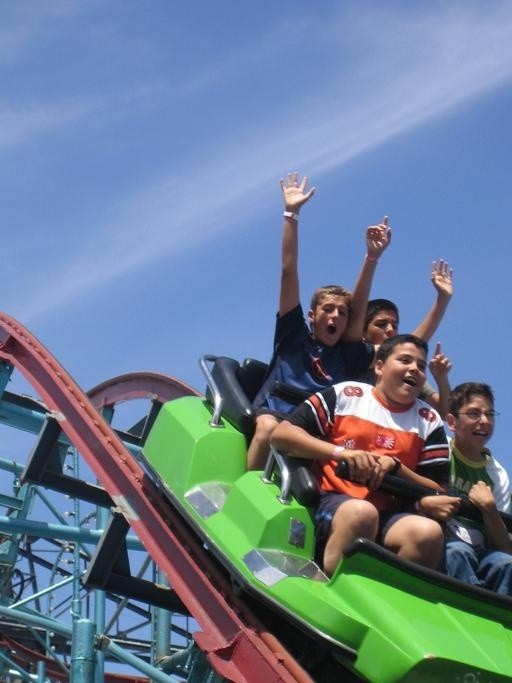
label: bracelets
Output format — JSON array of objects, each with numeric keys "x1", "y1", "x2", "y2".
[
  {"x1": 332, "y1": 447, "x2": 345, "y2": 458},
  {"x1": 284, "y1": 210, "x2": 299, "y2": 220},
  {"x1": 365, "y1": 253, "x2": 377, "y2": 262}
]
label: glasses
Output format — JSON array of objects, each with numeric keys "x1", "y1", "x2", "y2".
[{"x1": 456, "y1": 410, "x2": 500, "y2": 419}]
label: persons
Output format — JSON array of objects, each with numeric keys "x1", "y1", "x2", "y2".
[
  {"x1": 345, "y1": 212, "x2": 454, "y2": 422},
  {"x1": 268, "y1": 335, "x2": 452, "y2": 578},
  {"x1": 412, "y1": 382, "x2": 512, "y2": 597},
  {"x1": 246, "y1": 169, "x2": 454, "y2": 472}
]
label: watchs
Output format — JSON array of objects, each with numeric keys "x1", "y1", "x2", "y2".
[{"x1": 389, "y1": 456, "x2": 401, "y2": 475}]
